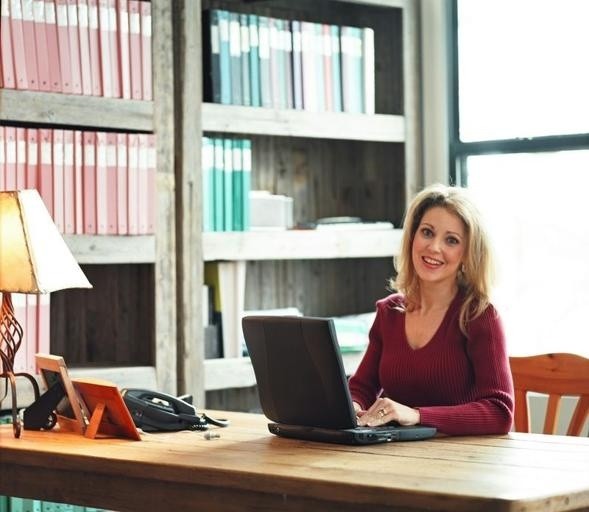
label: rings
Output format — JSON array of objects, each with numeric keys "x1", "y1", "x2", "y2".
[{"x1": 378, "y1": 408, "x2": 386, "y2": 416}]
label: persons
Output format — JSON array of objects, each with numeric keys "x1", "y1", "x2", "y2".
[{"x1": 340, "y1": 181, "x2": 516, "y2": 438}]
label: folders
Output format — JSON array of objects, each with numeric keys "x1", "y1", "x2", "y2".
[
  {"x1": 202, "y1": 9, "x2": 376, "y2": 115},
  {"x1": 0, "y1": 0, "x2": 154, "y2": 100},
  {"x1": 0, "y1": 127, "x2": 155, "y2": 237},
  {"x1": 202, "y1": 135, "x2": 250, "y2": 234}
]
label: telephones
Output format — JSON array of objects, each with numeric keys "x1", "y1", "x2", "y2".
[{"x1": 123, "y1": 389, "x2": 201, "y2": 432}]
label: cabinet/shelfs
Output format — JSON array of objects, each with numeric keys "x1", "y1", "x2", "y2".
[
  {"x1": 0, "y1": 1, "x2": 176, "y2": 409},
  {"x1": 173, "y1": 2, "x2": 422, "y2": 411}
]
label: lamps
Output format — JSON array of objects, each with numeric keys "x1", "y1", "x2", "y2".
[{"x1": 0, "y1": 186, "x2": 93, "y2": 438}]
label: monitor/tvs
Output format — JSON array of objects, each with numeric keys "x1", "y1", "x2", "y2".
[{"x1": 241, "y1": 315, "x2": 437, "y2": 446}]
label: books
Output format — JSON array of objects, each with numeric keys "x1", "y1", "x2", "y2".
[{"x1": 0, "y1": 413, "x2": 113, "y2": 511}]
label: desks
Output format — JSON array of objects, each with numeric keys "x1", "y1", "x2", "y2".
[{"x1": 0, "y1": 409, "x2": 589, "y2": 511}]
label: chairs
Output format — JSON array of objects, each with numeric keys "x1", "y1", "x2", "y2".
[{"x1": 509, "y1": 354, "x2": 589, "y2": 436}]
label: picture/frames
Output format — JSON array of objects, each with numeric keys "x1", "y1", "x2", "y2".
[{"x1": 23, "y1": 353, "x2": 141, "y2": 440}]
